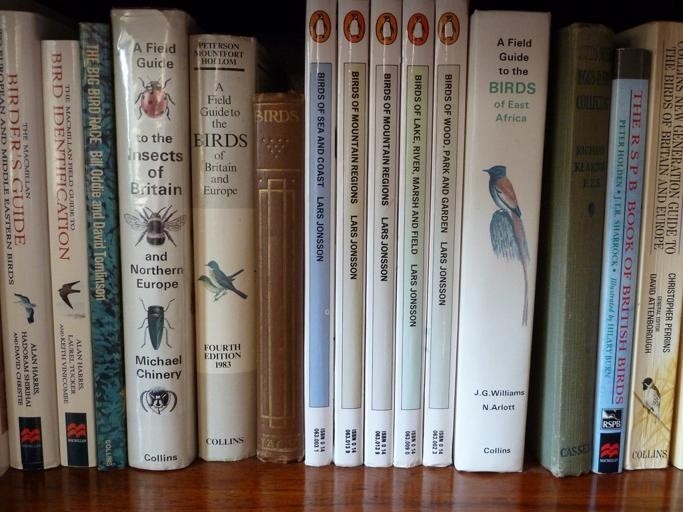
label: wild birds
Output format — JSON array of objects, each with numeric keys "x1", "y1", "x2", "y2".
[
  {"x1": 642, "y1": 377, "x2": 661, "y2": 416},
  {"x1": 57, "y1": 280, "x2": 80, "y2": 309},
  {"x1": 198, "y1": 268, "x2": 245, "y2": 300},
  {"x1": 482, "y1": 165, "x2": 531, "y2": 326},
  {"x1": 203, "y1": 260, "x2": 248, "y2": 300},
  {"x1": 13, "y1": 293, "x2": 37, "y2": 324}
]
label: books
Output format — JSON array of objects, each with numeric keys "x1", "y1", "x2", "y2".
[{"x1": 2, "y1": 1, "x2": 682, "y2": 479}]
